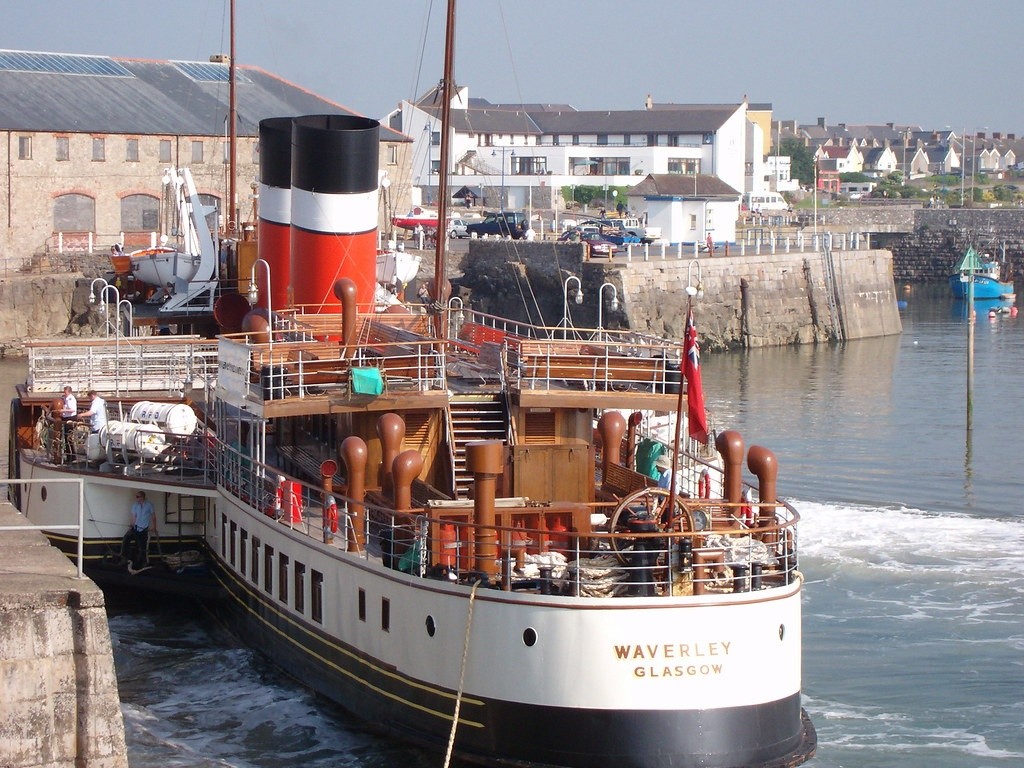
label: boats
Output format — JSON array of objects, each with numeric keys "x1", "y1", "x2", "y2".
[
  {"x1": 948, "y1": 231, "x2": 1014, "y2": 301},
  {"x1": 1000, "y1": 292, "x2": 1019, "y2": 300},
  {"x1": 1, "y1": 1, "x2": 817, "y2": 768}
]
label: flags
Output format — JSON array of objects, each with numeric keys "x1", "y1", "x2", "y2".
[{"x1": 679, "y1": 298, "x2": 708, "y2": 444}]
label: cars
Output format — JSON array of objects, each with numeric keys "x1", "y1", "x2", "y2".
[
  {"x1": 557, "y1": 217, "x2": 654, "y2": 258},
  {"x1": 448, "y1": 218, "x2": 472, "y2": 239}
]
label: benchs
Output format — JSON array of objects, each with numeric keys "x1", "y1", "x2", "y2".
[
  {"x1": 447, "y1": 320, "x2": 540, "y2": 387},
  {"x1": 592, "y1": 428, "x2": 627, "y2": 470},
  {"x1": 522, "y1": 356, "x2": 663, "y2": 390},
  {"x1": 274, "y1": 445, "x2": 347, "y2": 510},
  {"x1": 390, "y1": 478, "x2": 452, "y2": 529},
  {"x1": 338, "y1": 316, "x2": 431, "y2": 360},
  {"x1": 292, "y1": 357, "x2": 437, "y2": 395},
  {"x1": 600, "y1": 462, "x2": 658, "y2": 524},
  {"x1": 576, "y1": 344, "x2": 638, "y2": 392},
  {"x1": 253, "y1": 341, "x2": 340, "y2": 373},
  {"x1": 456, "y1": 341, "x2": 502, "y2": 386},
  {"x1": 674, "y1": 498, "x2": 730, "y2": 531},
  {"x1": 292, "y1": 314, "x2": 430, "y2": 342},
  {"x1": 521, "y1": 339, "x2": 606, "y2": 363}
]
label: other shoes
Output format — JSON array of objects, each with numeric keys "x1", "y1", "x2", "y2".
[
  {"x1": 64, "y1": 458, "x2": 77, "y2": 464},
  {"x1": 118, "y1": 557, "x2": 127, "y2": 566}
]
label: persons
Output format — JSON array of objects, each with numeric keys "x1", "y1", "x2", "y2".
[
  {"x1": 513, "y1": 223, "x2": 580, "y2": 241},
  {"x1": 55, "y1": 387, "x2": 78, "y2": 458},
  {"x1": 706, "y1": 233, "x2": 714, "y2": 251},
  {"x1": 77, "y1": 389, "x2": 108, "y2": 468},
  {"x1": 1011, "y1": 192, "x2": 1023, "y2": 206},
  {"x1": 750, "y1": 199, "x2": 793, "y2": 215},
  {"x1": 598, "y1": 200, "x2": 628, "y2": 219},
  {"x1": 928, "y1": 195, "x2": 944, "y2": 209},
  {"x1": 116, "y1": 491, "x2": 157, "y2": 570},
  {"x1": 652, "y1": 454, "x2": 678, "y2": 529},
  {"x1": 412, "y1": 223, "x2": 430, "y2": 305},
  {"x1": 428, "y1": 193, "x2": 473, "y2": 209}
]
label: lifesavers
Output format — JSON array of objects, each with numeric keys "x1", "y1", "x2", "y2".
[
  {"x1": 698, "y1": 468, "x2": 711, "y2": 501},
  {"x1": 739, "y1": 490, "x2": 753, "y2": 530},
  {"x1": 320, "y1": 496, "x2": 338, "y2": 540}
]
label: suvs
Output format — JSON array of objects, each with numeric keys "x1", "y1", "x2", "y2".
[{"x1": 466, "y1": 211, "x2": 530, "y2": 240}]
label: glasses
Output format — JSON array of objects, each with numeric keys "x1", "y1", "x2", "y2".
[{"x1": 136, "y1": 495, "x2": 142, "y2": 498}]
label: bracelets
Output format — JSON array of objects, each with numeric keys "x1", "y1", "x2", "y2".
[{"x1": 658, "y1": 505, "x2": 662, "y2": 509}]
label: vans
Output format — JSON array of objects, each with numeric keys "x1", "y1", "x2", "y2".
[{"x1": 744, "y1": 191, "x2": 789, "y2": 215}]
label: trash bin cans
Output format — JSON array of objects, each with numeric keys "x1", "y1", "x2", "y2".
[{"x1": 662, "y1": 363, "x2": 683, "y2": 394}]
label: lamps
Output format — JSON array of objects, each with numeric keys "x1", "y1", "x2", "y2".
[
  {"x1": 247, "y1": 258, "x2": 273, "y2": 400},
  {"x1": 563, "y1": 275, "x2": 584, "y2": 340},
  {"x1": 447, "y1": 296, "x2": 465, "y2": 352},
  {"x1": 97, "y1": 284, "x2": 119, "y2": 336},
  {"x1": 114, "y1": 299, "x2": 132, "y2": 337},
  {"x1": 685, "y1": 259, "x2": 704, "y2": 300},
  {"x1": 598, "y1": 283, "x2": 619, "y2": 340},
  {"x1": 88, "y1": 278, "x2": 110, "y2": 338}
]
